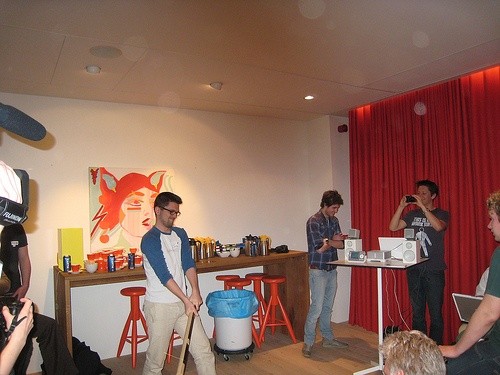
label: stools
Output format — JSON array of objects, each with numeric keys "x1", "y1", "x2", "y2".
[
  {"x1": 116, "y1": 286, "x2": 148, "y2": 369},
  {"x1": 167, "y1": 329, "x2": 183, "y2": 364},
  {"x1": 212, "y1": 273, "x2": 298, "y2": 351}
]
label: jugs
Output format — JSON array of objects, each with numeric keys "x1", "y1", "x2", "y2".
[
  {"x1": 189, "y1": 237, "x2": 216, "y2": 263},
  {"x1": 245, "y1": 234, "x2": 272, "y2": 256}
]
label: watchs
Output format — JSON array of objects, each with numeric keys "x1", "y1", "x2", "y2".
[
  {"x1": 423, "y1": 208, "x2": 430, "y2": 214},
  {"x1": 325, "y1": 240, "x2": 329, "y2": 244}
]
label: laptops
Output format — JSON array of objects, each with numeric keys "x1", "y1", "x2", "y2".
[
  {"x1": 451, "y1": 293, "x2": 484, "y2": 323},
  {"x1": 378, "y1": 237, "x2": 406, "y2": 259}
]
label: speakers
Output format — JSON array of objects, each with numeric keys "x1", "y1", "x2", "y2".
[
  {"x1": 344, "y1": 228, "x2": 361, "y2": 260},
  {"x1": 403, "y1": 228, "x2": 418, "y2": 265}
]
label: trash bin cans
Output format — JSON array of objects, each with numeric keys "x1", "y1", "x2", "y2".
[{"x1": 208, "y1": 289, "x2": 256, "y2": 362}]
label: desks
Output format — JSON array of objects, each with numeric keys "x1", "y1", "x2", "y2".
[
  {"x1": 53, "y1": 250, "x2": 311, "y2": 361},
  {"x1": 326, "y1": 257, "x2": 431, "y2": 375}
]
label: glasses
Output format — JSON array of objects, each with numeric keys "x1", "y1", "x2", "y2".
[{"x1": 157, "y1": 205, "x2": 181, "y2": 216}]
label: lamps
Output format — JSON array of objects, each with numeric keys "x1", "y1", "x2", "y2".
[
  {"x1": 86, "y1": 65, "x2": 101, "y2": 74},
  {"x1": 209, "y1": 80, "x2": 224, "y2": 90}
]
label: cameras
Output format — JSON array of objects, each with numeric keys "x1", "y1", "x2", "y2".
[
  {"x1": 0, "y1": 295, "x2": 34, "y2": 331},
  {"x1": 406, "y1": 196, "x2": 417, "y2": 202},
  {"x1": 349, "y1": 251, "x2": 366, "y2": 261}
]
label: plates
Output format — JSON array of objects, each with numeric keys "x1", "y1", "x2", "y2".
[{"x1": 68, "y1": 270, "x2": 83, "y2": 275}]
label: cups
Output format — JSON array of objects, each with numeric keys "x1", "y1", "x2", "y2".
[
  {"x1": 216, "y1": 244, "x2": 245, "y2": 257},
  {"x1": 85, "y1": 247, "x2": 143, "y2": 274},
  {"x1": 70, "y1": 265, "x2": 81, "y2": 273}
]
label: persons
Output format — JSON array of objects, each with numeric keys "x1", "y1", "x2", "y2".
[
  {"x1": 140, "y1": 192, "x2": 216, "y2": 375},
  {"x1": 302, "y1": 189, "x2": 349, "y2": 356},
  {"x1": 0, "y1": 223, "x2": 81, "y2": 375},
  {"x1": 455, "y1": 266, "x2": 490, "y2": 342},
  {"x1": 388, "y1": 180, "x2": 451, "y2": 346},
  {"x1": 437, "y1": 191, "x2": 500, "y2": 375},
  {"x1": 378, "y1": 330, "x2": 447, "y2": 375}
]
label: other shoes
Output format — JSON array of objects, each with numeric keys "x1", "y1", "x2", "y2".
[
  {"x1": 322, "y1": 338, "x2": 349, "y2": 348},
  {"x1": 302, "y1": 343, "x2": 312, "y2": 357}
]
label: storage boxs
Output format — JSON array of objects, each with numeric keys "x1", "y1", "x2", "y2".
[{"x1": 58, "y1": 227, "x2": 85, "y2": 270}]
